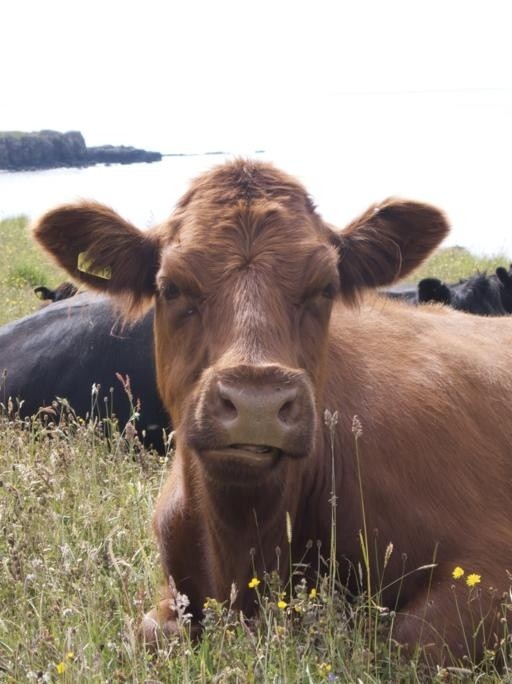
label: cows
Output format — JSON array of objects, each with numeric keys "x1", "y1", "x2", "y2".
[
  {"x1": 0, "y1": 264, "x2": 511, "y2": 460},
  {"x1": 34, "y1": 155, "x2": 512, "y2": 679},
  {"x1": 34, "y1": 280, "x2": 76, "y2": 302}
]
